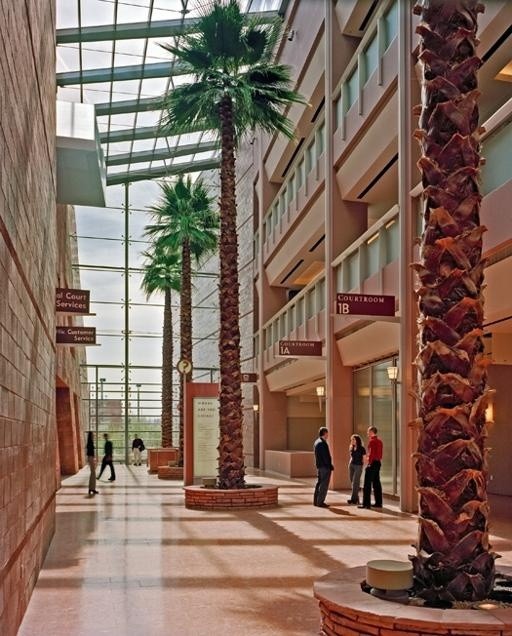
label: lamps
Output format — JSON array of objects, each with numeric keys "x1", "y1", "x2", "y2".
[
  {"x1": 316, "y1": 385, "x2": 326, "y2": 397},
  {"x1": 386, "y1": 366, "x2": 401, "y2": 385},
  {"x1": 253, "y1": 404, "x2": 259, "y2": 413}
]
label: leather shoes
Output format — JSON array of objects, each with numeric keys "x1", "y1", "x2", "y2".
[{"x1": 356, "y1": 504, "x2": 383, "y2": 510}]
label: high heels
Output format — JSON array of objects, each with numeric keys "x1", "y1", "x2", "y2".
[{"x1": 88, "y1": 489, "x2": 99, "y2": 495}]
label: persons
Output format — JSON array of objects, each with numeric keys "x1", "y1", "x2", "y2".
[
  {"x1": 85, "y1": 430, "x2": 99, "y2": 494},
  {"x1": 95, "y1": 433, "x2": 116, "y2": 481},
  {"x1": 357, "y1": 425, "x2": 384, "y2": 509},
  {"x1": 313, "y1": 426, "x2": 334, "y2": 507},
  {"x1": 131, "y1": 433, "x2": 146, "y2": 466},
  {"x1": 346, "y1": 434, "x2": 367, "y2": 504}
]
